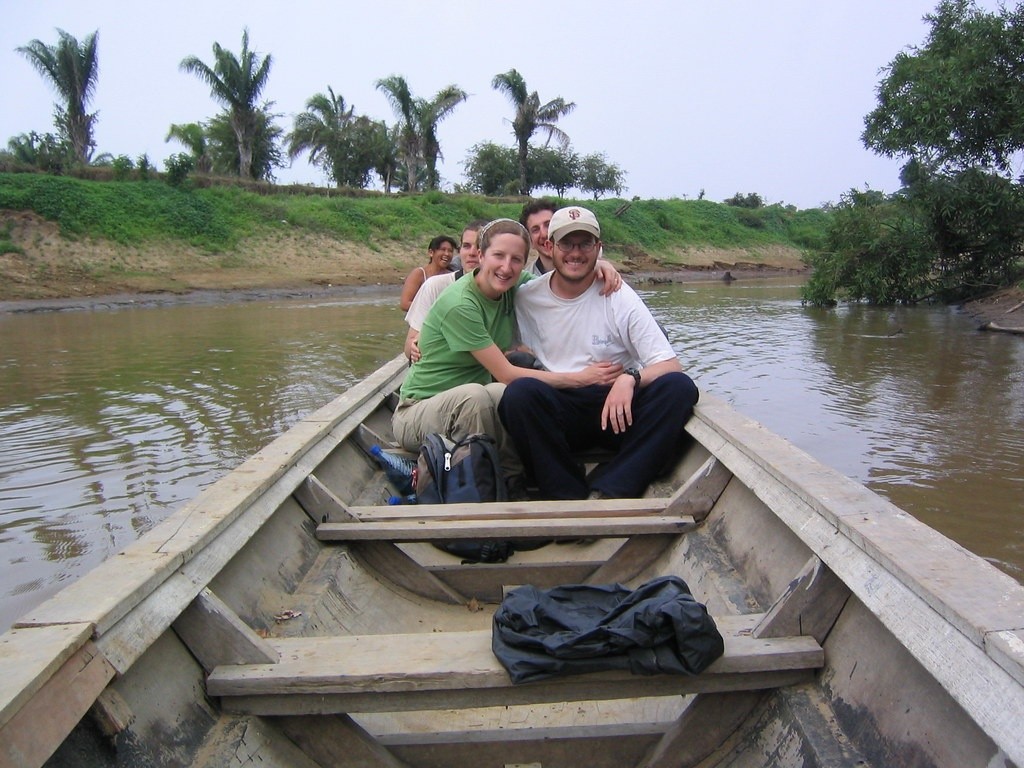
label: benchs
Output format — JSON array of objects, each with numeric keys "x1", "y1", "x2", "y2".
[
  {"x1": 207, "y1": 613, "x2": 824, "y2": 716},
  {"x1": 381, "y1": 442, "x2": 418, "y2": 460},
  {"x1": 315, "y1": 498, "x2": 695, "y2": 540}
]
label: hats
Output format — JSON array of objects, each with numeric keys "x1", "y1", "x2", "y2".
[{"x1": 547, "y1": 206, "x2": 600, "y2": 244}]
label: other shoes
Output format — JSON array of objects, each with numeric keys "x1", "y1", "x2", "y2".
[{"x1": 586, "y1": 489, "x2": 613, "y2": 545}]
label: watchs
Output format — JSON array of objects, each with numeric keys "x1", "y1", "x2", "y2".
[{"x1": 624, "y1": 367, "x2": 641, "y2": 387}]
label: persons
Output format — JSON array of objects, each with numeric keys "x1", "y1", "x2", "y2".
[
  {"x1": 411, "y1": 206, "x2": 699, "y2": 544},
  {"x1": 519, "y1": 196, "x2": 560, "y2": 277},
  {"x1": 400, "y1": 235, "x2": 459, "y2": 312},
  {"x1": 404, "y1": 220, "x2": 539, "y2": 382},
  {"x1": 448, "y1": 244, "x2": 462, "y2": 271},
  {"x1": 390, "y1": 217, "x2": 624, "y2": 550}
]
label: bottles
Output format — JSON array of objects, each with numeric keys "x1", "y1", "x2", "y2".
[
  {"x1": 388, "y1": 493, "x2": 417, "y2": 506},
  {"x1": 370, "y1": 444, "x2": 417, "y2": 493}
]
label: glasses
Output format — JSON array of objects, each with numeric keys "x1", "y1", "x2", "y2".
[{"x1": 555, "y1": 237, "x2": 597, "y2": 253}]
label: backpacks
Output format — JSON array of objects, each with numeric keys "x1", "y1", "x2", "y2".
[{"x1": 415, "y1": 429, "x2": 512, "y2": 564}]
